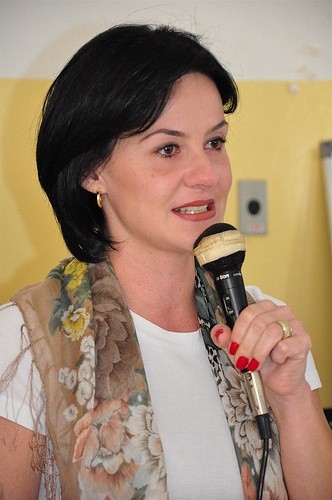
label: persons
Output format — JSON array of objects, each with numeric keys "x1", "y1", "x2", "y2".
[{"x1": 0, "y1": 26, "x2": 332, "y2": 500}]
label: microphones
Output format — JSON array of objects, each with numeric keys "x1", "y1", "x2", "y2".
[{"x1": 194, "y1": 222, "x2": 273, "y2": 441}]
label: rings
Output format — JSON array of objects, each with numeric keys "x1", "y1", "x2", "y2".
[{"x1": 275, "y1": 320, "x2": 294, "y2": 340}]
